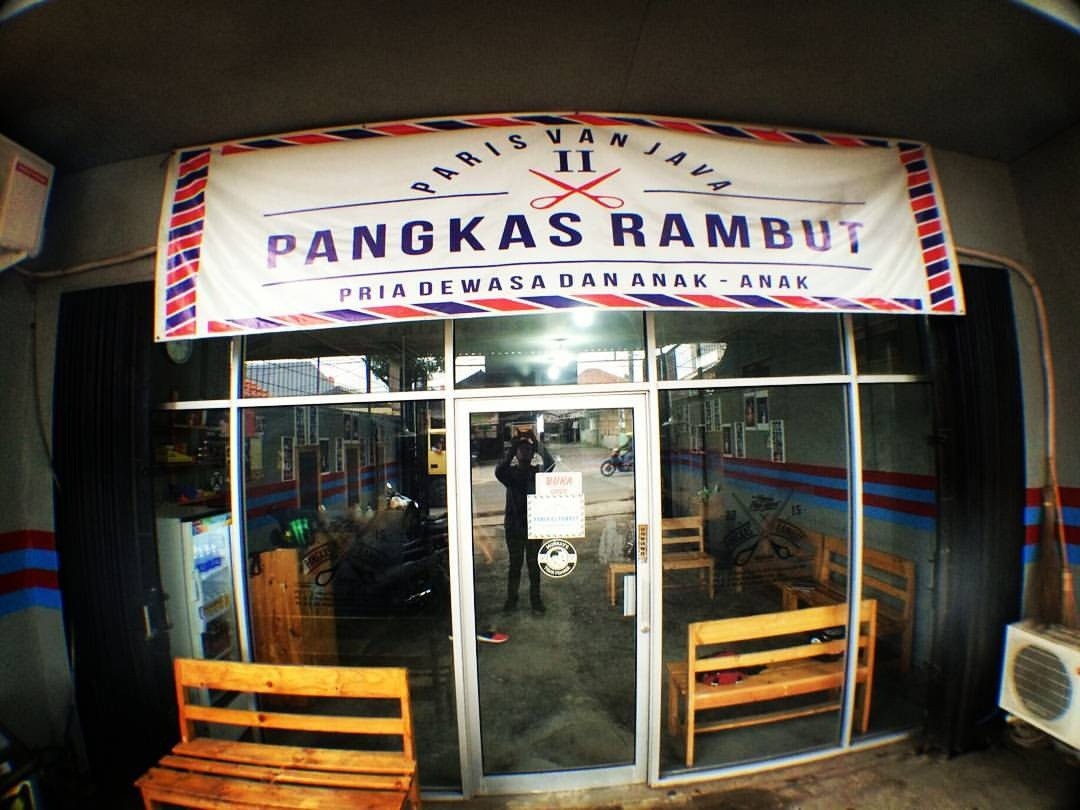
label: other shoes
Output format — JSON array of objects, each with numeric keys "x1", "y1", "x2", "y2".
[
  {"x1": 530, "y1": 597, "x2": 546, "y2": 614},
  {"x1": 504, "y1": 596, "x2": 519, "y2": 609}
]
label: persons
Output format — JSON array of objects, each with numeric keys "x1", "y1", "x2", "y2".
[
  {"x1": 619, "y1": 433, "x2": 633, "y2": 464},
  {"x1": 493, "y1": 427, "x2": 555, "y2": 613}
]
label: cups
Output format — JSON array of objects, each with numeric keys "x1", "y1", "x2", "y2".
[{"x1": 185, "y1": 411, "x2": 208, "y2": 425}]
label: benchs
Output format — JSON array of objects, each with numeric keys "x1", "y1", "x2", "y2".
[
  {"x1": 606, "y1": 516, "x2": 715, "y2": 607},
  {"x1": 134, "y1": 657, "x2": 421, "y2": 810},
  {"x1": 669, "y1": 599, "x2": 877, "y2": 764},
  {"x1": 785, "y1": 535, "x2": 917, "y2": 672}
]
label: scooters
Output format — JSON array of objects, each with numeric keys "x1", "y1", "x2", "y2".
[
  {"x1": 599, "y1": 444, "x2": 634, "y2": 476},
  {"x1": 297, "y1": 481, "x2": 448, "y2": 637}
]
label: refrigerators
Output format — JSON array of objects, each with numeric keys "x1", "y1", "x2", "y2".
[{"x1": 154, "y1": 505, "x2": 251, "y2": 743}]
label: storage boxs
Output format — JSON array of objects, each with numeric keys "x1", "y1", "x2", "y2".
[{"x1": 687, "y1": 495, "x2": 721, "y2": 519}]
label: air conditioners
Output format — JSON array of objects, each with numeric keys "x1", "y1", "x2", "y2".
[{"x1": 998, "y1": 618, "x2": 1080, "y2": 750}]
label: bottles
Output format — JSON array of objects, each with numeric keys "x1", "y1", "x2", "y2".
[{"x1": 193, "y1": 539, "x2": 231, "y2": 659}]
